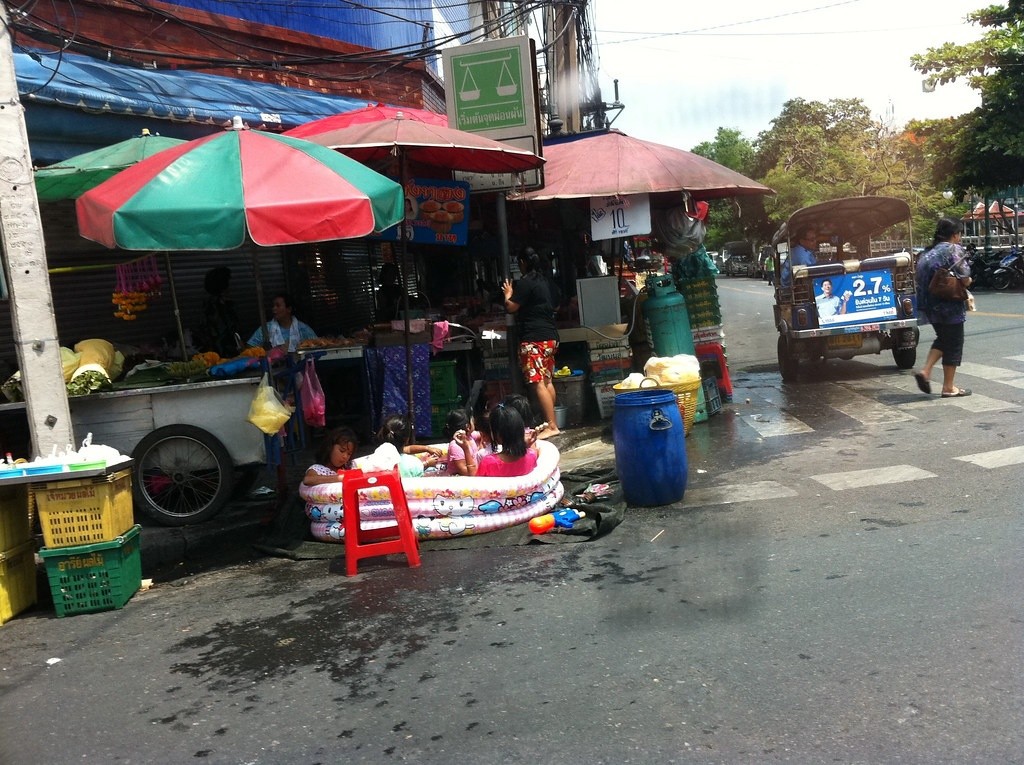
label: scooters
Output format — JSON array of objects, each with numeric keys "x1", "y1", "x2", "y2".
[{"x1": 963, "y1": 240, "x2": 1024, "y2": 291}]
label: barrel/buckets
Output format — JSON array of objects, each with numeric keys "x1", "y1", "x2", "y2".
[
  {"x1": 613, "y1": 389, "x2": 687, "y2": 507},
  {"x1": 554, "y1": 406, "x2": 567, "y2": 429},
  {"x1": 399, "y1": 310, "x2": 423, "y2": 320},
  {"x1": 553, "y1": 376, "x2": 586, "y2": 424}
]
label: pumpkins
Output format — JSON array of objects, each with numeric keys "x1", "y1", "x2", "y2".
[{"x1": 192, "y1": 347, "x2": 265, "y2": 364}]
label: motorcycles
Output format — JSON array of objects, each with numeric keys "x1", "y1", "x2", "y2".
[{"x1": 766, "y1": 194, "x2": 922, "y2": 383}]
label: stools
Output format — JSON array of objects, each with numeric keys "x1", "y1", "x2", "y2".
[{"x1": 342, "y1": 465, "x2": 419, "y2": 577}]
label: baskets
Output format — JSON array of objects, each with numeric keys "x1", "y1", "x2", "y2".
[{"x1": 613, "y1": 376, "x2": 702, "y2": 438}]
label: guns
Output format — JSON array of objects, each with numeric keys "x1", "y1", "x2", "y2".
[{"x1": 528, "y1": 506, "x2": 587, "y2": 536}]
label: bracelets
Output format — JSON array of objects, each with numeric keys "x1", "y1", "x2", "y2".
[{"x1": 466, "y1": 464, "x2": 476, "y2": 467}]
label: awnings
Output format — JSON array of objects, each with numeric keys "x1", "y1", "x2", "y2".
[{"x1": 14, "y1": 44, "x2": 408, "y2": 162}]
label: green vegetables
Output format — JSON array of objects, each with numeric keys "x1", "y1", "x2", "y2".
[{"x1": 65, "y1": 370, "x2": 111, "y2": 395}]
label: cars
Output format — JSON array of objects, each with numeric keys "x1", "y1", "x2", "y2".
[{"x1": 706, "y1": 240, "x2": 779, "y2": 278}]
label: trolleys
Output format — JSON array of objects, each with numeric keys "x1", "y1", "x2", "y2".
[{"x1": 0, "y1": 364, "x2": 298, "y2": 530}]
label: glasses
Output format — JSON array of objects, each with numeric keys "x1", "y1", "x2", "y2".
[{"x1": 803, "y1": 237, "x2": 816, "y2": 242}]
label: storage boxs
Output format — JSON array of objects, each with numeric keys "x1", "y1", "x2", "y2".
[
  {"x1": 33, "y1": 468, "x2": 134, "y2": 549},
  {"x1": 589, "y1": 338, "x2": 640, "y2": 419},
  {"x1": 39, "y1": 524, "x2": 143, "y2": 618},
  {"x1": 426, "y1": 362, "x2": 463, "y2": 401},
  {"x1": 431, "y1": 402, "x2": 461, "y2": 436},
  {"x1": 0, "y1": 486, "x2": 37, "y2": 624},
  {"x1": 681, "y1": 277, "x2": 730, "y2": 416}
]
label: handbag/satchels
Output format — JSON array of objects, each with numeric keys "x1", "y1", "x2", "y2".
[
  {"x1": 247, "y1": 372, "x2": 295, "y2": 436},
  {"x1": 295, "y1": 354, "x2": 326, "y2": 428},
  {"x1": 965, "y1": 289, "x2": 976, "y2": 312},
  {"x1": 928, "y1": 250, "x2": 970, "y2": 301}
]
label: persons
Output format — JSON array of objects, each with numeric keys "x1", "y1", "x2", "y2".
[
  {"x1": 245, "y1": 285, "x2": 319, "y2": 434},
  {"x1": 779, "y1": 224, "x2": 817, "y2": 291},
  {"x1": 476, "y1": 403, "x2": 537, "y2": 477},
  {"x1": 914, "y1": 218, "x2": 973, "y2": 397},
  {"x1": 303, "y1": 426, "x2": 357, "y2": 486},
  {"x1": 815, "y1": 280, "x2": 850, "y2": 316},
  {"x1": 442, "y1": 407, "x2": 478, "y2": 476},
  {"x1": 501, "y1": 245, "x2": 560, "y2": 439},
  {"x1": 375, "y1": 414, "x2": 443, "y2": 471},
  {"x1": 501, "y1": 393, "x2": 548, "y2": 453},
  {"x1": 764, "y1": 253, "x2": 775, "y2": 285}
]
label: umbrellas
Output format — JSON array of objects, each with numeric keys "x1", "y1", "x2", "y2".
[
  {"x1": 279, "y1": 103, "x2": 448, "y2": 138},
  {"x1": 301, "y1": 111, "x2": 547, "y2": 446},
  {"x1": 507, "y1": 132, "x2": 777, "y2": 297},
  {"x1": 33, "y1": 128, "x2": 190, "y2": 363},
  {"x1": 75, "y1": 115, "x2": 404, "y2": 342}
]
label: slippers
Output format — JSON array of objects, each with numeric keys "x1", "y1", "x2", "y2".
[
  {"x1": 538, "y1": 429, "x2": 559, "y2": 439},
  {"x1": 915, "y1": 373, "x2": 931, "y2": 394},
  {"x1": 942, "y1": 386, "x2": 972, "y2": 396}
]
